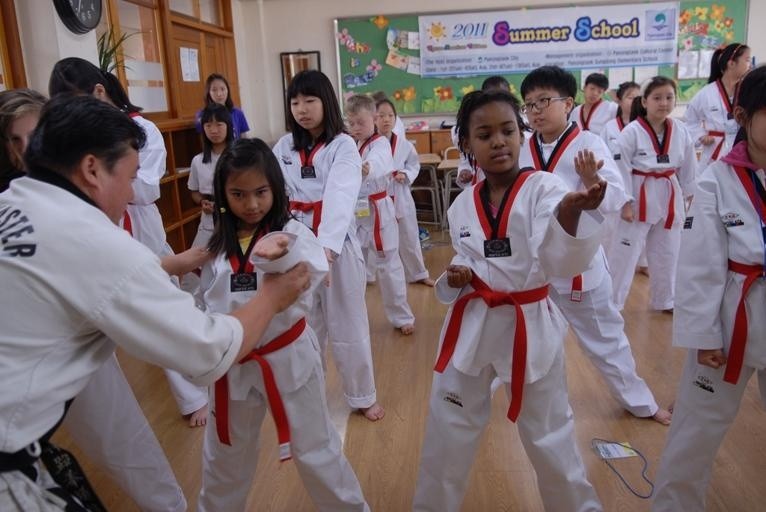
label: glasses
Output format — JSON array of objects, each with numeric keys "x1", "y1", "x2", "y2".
[{"x1": 519, "y1": 97, "x2": 565, "y2": 114}]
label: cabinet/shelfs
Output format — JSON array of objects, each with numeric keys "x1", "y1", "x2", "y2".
[
  {"x1": 431, "y1": 130, "x2": 461, "y2": 159},
  {"x1": 139, "y1": 115, "x2": 200, "y2": 248},
  {"x1": 403, "y1": 130, "x2": 430, "y2": 154},
  {"x1": 282, "y1": 55, "x2": 311, "y2": 85}
]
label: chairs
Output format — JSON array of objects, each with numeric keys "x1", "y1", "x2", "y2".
[{"x1": 412, "y1": 146, "x2": 465, "y2": 228}]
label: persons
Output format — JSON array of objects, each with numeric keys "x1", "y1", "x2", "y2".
[
  {"x1": 346, "y1": 90, "x2": 436, "y2": 335},
  {"x1": 682, "y1": 45, "x2": 764, "y2": 510},
  {"x1": 413, "y1": 86, "x2": 608, "y2": 510},
  {"x1": 449, "y1": 65, "x2": 687, "y2": 425},
  {"x1": 193, "y1": 136, "x2": 374, "y2": 511},
  {"x1": 272, "y1": 70, "x2": 386, "y2": 422},
  {"x1": 1, "y1": 72, "x2": 311, "y2": 511}
]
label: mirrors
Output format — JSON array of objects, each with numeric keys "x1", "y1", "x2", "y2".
[{"x1": 278, "y1": 51, "x2": 321, "y2": 131}]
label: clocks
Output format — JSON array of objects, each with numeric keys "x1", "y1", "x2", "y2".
[{"x1": 52, "y1": 0, "x2": 102, "y2": 34}]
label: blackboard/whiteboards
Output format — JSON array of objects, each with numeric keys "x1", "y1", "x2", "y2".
[{"x1": 334, "y1": 1, "x2": 750, "y2": 120}]
